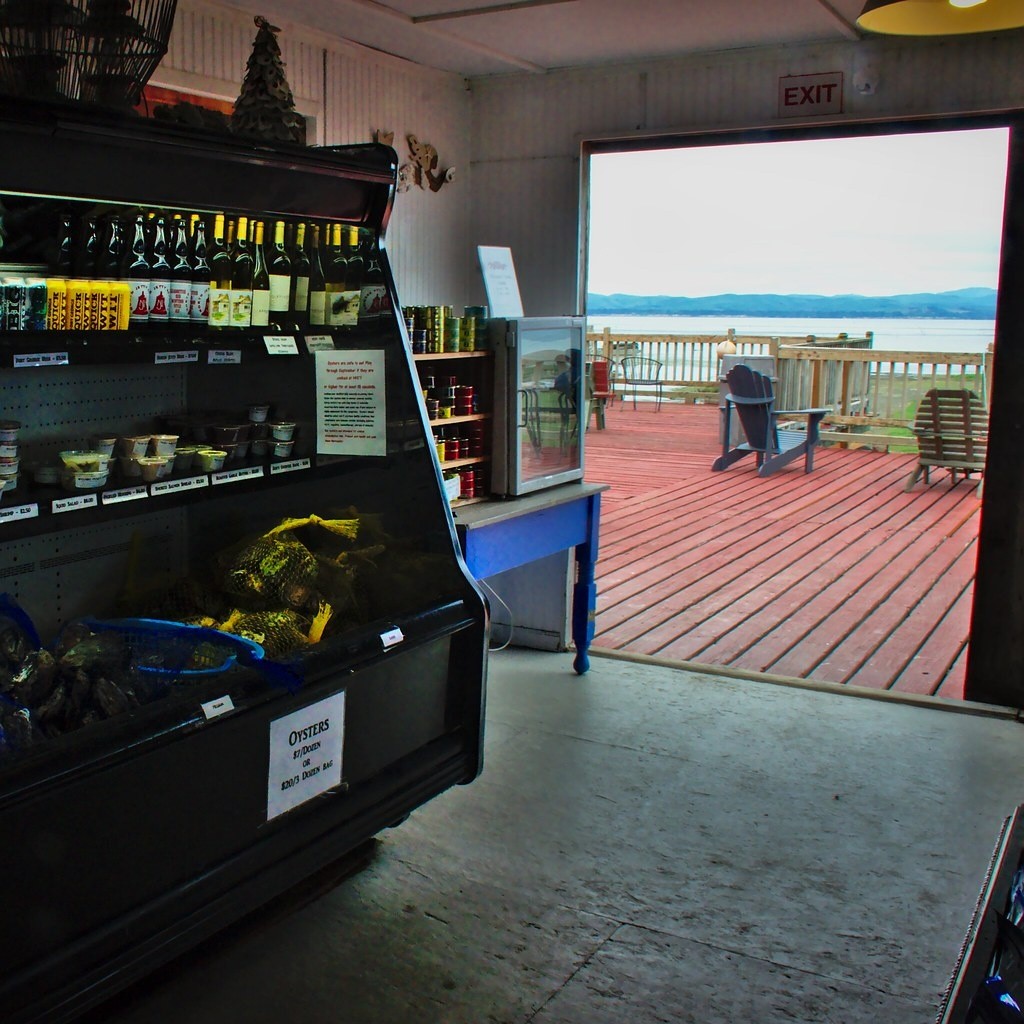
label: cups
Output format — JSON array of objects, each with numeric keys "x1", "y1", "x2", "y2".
[
  {"x1": 123, "y1": 404, "x2": 295, "y2": 482},
  {"x1": 0, "y1": 420, "x2": 22, "y2": 501},
  {"x1": 92, "y1": 435, "x2": 117, "y2": 477}
]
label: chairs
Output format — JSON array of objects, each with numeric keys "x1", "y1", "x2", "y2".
[
  {"x1": 574, "y1": 354, "x2": 616, "y2": 431},
  {"x1": 621, "y1": 357, "x2": 664, "y2": 410},
  {"x1": 710, "y1": 364, "x2": 833, "y2": 480},
  {"x1": 906, "y1": 389, "x2": 992, "y2": 492}
]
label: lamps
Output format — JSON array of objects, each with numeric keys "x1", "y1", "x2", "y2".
[{"x1": 856, "y1": 0, "x2": 1024, "y2": 39}]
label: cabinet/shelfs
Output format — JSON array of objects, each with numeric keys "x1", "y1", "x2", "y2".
[
  {"x1": 414, "y1": 349, "x2": 494, "y2": 508},
  {"x1": 2, "y1": 101, "x2": 493, "y2": 1023}
]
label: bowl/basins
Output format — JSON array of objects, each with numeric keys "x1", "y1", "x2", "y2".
[
  {"x1": 60, "y1": 452, "x2": 109, "y2": 492},
  {"x1": 120, "y1": 458, "x2": 167, "y2": 482}
]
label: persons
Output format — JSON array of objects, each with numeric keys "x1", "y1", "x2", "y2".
[{"x1": 554, "y1": 355, "x2": 572, "y2": 393}]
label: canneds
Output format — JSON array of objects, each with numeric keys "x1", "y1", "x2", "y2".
[
  {"x1": 421, "y1": 376, "x2": 490, "y2": 499},
  {"x1": 401, "y1": 304, "x2": 490, "y2": 353},
  {"x1": 0, "y1": 277, "x2": 131, "y2": 331}
]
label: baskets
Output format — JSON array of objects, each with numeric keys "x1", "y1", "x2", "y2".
[{"x1": 66, "y1": 616, "x2": 266, "y2": 698}]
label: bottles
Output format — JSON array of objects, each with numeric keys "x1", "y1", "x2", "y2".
[
  {"x1": 34, "y1": 464, "x2": 61, "y2": 484},
  {"x1": 207, "y1": 215, "x2": 391, "y2": 331},
  {"x1": 50, "y1": 213, "x2": 212, "y2": 321}
]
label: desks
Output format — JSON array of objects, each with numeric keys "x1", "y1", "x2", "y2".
[{"x1": 454, "y1": 482, "x2": 609, "y2": 677}]
